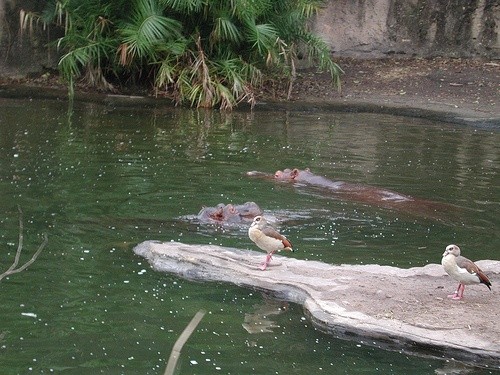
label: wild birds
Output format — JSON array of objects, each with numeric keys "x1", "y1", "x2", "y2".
[
  {"x1": 441, "y1": 244, "x2": 492, "y2": 299},
  {"x1": 248, "y1": 216, "x2": 294, "y2": 271}
]
